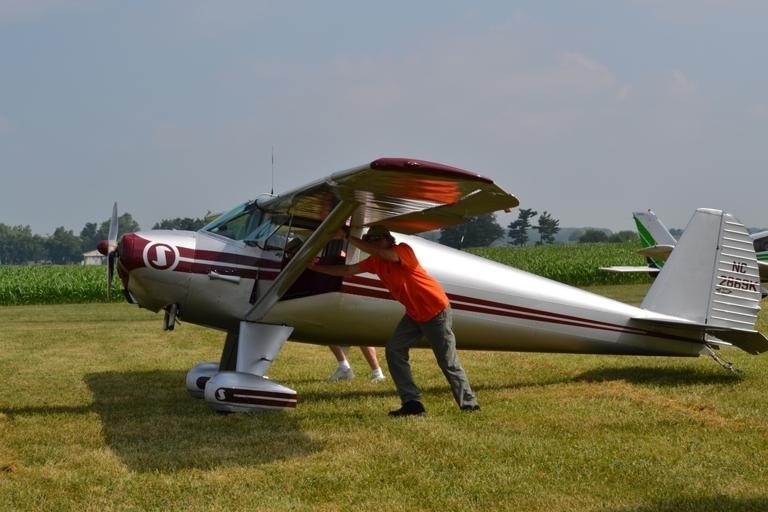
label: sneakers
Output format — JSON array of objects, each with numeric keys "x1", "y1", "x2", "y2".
[
  {"x1": 388, "y1": 400, "x2": 428, "y2": 418},
  {"x1": 369, "y1": 375, "x2": 386, "y2": 384},
  {"x1": 327, "y1": 366, "x2": 356, "y2": 382}
]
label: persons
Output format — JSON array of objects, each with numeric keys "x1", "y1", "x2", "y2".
[
  {"x1": 276, "y1": 224, "x2": 388, "y2": 381},
  {"x1": 309, "y1": 225, "x2": 482, "y2": 416}
]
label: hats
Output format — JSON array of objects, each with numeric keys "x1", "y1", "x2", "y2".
[{"x1": 361, "y1": 225, "x2": 395, "y2": 243}]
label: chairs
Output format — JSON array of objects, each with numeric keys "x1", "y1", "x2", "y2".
[{"x1": 319, "y1": 239, "x2": 346, "y2": 258}]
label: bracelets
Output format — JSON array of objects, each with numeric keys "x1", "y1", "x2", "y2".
[{"x1": 343, "y1": 233, "x2": 349, "y2": 241}]
